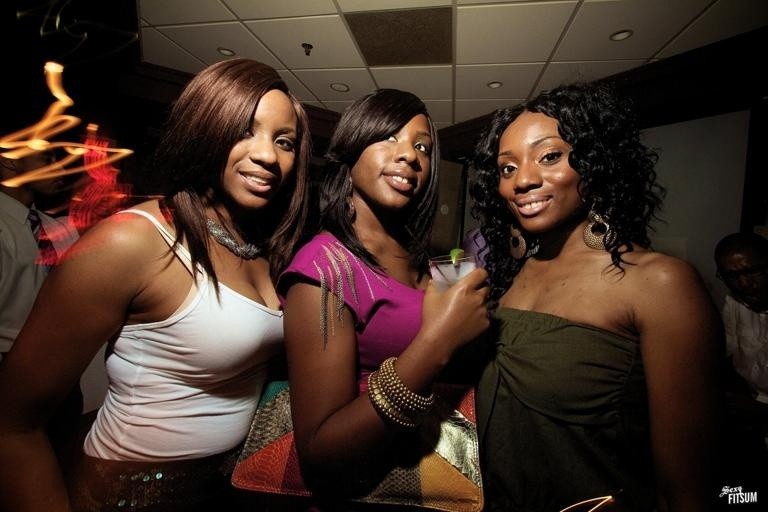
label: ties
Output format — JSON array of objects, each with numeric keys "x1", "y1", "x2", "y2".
[{"x1": 27, "y1": 203, "x2": 59, "y2": 275}]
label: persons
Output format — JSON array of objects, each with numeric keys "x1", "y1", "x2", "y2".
[
  {"x1": 1, "y1": 57, "x2": 315, "y2": 512},
  {"x1": 274, "y1": 87, "x2": 492, "y2": 512},
  {"x1": 0, "y1": 124, "x2": 84, "y2": 447},
  {"x1": 713, "y1": 232, "x2": 768, "y2": 407},
  {"x1": 460, "y1": 76, "x2": 722, "y2": 511}
]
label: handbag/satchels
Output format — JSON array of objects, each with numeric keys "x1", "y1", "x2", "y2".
[{"x1": 232, "y1": 368, "x2": 486, "y2": 511}]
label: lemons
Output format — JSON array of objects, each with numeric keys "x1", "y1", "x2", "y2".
[{"x1": 450, "y1": 248, "x2": 466, "y2": 268}]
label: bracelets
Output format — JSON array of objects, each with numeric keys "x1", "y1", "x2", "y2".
[{"x1": 366, "y1": 356, "x2": 438, "y2": 429}]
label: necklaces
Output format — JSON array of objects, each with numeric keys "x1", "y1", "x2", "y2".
[{"x1": 206, "y1": 218, "x2": 263, "y2": 261}]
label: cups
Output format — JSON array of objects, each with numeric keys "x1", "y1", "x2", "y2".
[{"x1": 428, "y1": 253, "x2": 479, "y2": 289}]
